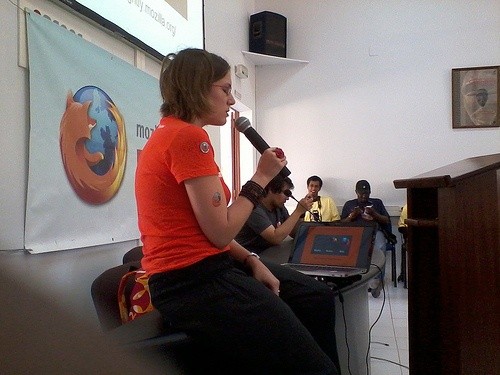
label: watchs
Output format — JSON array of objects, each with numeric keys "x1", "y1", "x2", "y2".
[{"x1": 244, "y1": 252, "x2": 260, "y2": 267}]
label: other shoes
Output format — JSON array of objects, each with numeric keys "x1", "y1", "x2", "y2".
[{"x1": 369, "y1": 278, "x2": 380, "y2": 298}]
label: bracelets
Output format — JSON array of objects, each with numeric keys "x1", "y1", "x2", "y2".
[
  {"x1": 238, "y1": 181, "x2": 265, "y2": 210},
  {"x1": 348, "y1": 214, "x2": 355, "y2": 220}
]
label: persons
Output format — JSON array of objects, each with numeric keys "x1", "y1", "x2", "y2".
[
  {"x1": 340, "y1": 179, "x2": 391, "y2": 298},
  {"x1": 233, "y1": 174, "x2": 313, "y2": 254},
  {"x1": 398, "y1": 203, "x2": 409, "y2": 233},
  {"x1": 303, "y1": 175, "x2": 341, "y2": 222},
  {"x1": 136, "y1": 48, "x2": 341, "y2": 375}
]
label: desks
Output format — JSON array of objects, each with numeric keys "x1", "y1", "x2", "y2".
[{"x1": 256, "y1": 240, "x2": 386, "y2": 375}]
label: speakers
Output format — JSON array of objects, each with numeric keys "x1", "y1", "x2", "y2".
[{"x1": 250, "y1": 10, "x2": 287, "y2": 58}]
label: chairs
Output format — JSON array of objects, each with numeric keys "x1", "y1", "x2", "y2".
[
  {"x1": 91, "y1": 246, "x2": 188, "y2": 375},
  {"x1": 386, "y1": 234, "x2": 407, "y2": 289}
]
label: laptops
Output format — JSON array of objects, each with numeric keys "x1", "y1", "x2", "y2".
[{"x1": 278, "y1": 220, "x2": 377, "y2": 281}]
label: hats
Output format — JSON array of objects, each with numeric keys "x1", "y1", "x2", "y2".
[{"x1": 356, "y1": 180, "x2": 371, "y2": 194}]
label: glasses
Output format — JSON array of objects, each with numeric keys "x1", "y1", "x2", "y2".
[{"x1": 206, "y1": 83, "x2": 232, "y2": 96}]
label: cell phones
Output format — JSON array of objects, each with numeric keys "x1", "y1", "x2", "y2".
[{"x1": 365, "y1": 206, "x2": 372, "y2": 213}]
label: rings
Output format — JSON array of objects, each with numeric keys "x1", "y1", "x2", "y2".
[
  {"x1": 275, "y1": 148, "x2": 284, "y2": 159},
  {"x1": 277, "y1": 290, "x2": 280, "y2": 293}
]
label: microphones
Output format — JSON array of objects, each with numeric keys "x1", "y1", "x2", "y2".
[
  {"x1": 234, "y1": 116, "x2": 291, "y2": 179},
  {"x1": 283, "y1": 189, "x2": 321, "y2": 222}
]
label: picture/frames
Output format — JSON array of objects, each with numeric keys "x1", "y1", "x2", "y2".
[{"x1": 451, "y1": 66, "x2": 500, "y2": 128}]
